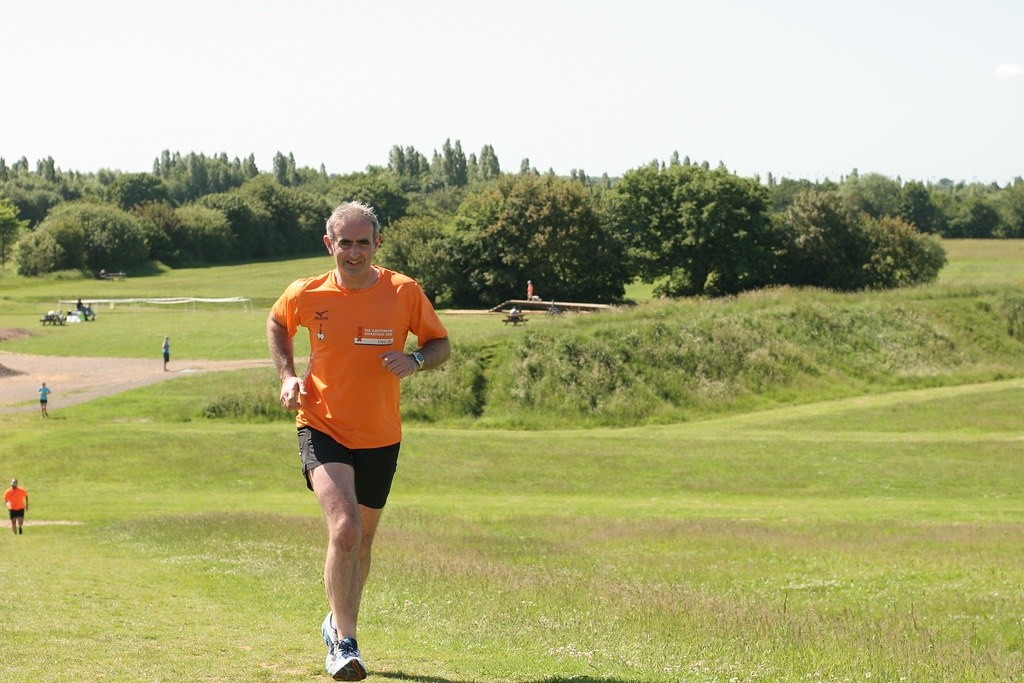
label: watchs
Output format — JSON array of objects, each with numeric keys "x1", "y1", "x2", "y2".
[{"x1": 409, "y1": 351, "x2": 425, "y2": 372}]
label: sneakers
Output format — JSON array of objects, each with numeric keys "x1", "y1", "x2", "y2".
[
  {"x1": 330, "y1": 636, "x2": 367, "y2": 681},
  {"x1": 321, "y1": 611, "x2": 339, "y2": 674}
]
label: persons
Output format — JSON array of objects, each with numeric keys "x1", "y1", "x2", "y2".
[
  {"x1": 508, "y1": 305, "x2": 521, "y2": 322},
  {"x1": 3, "y1": 478, "x2": 30, "y2": 535},
  {"x1": 39, "y1": 296, "x2": 96, "y2": 326},
  {"x1": 161, "y1": 336, "x2": 170, "y2": 373},
  {"x1": 37, "y1": 382, "x2": 50, "y2": 419},
  {"x1": 265, "y1": 202, "x2": 453, "y2": 682},
  {"x1": 526, "y1": 280, "x2": 534, "y2": 301}
]
